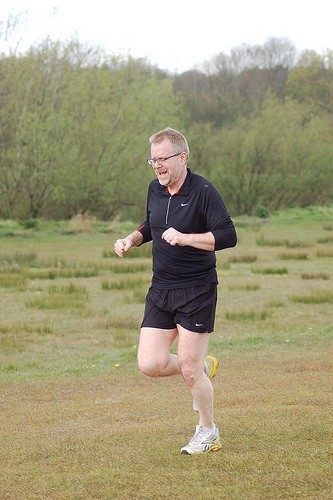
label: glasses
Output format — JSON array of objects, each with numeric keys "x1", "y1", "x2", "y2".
[{"x1": 147, "y1": 153, "x2": 181, "y2": 165}]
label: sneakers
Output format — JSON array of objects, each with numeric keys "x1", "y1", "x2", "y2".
[
  {"x1": 180, "y1": 424, "x2": 222, "y2": 455},
  {"x1": 193, "y1": 355, "x2": 217, "y2": 411}
]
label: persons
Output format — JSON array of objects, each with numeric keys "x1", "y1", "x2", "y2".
[{"x1": 110, "y1": 127, "x2": 242, "y2": 456}]
